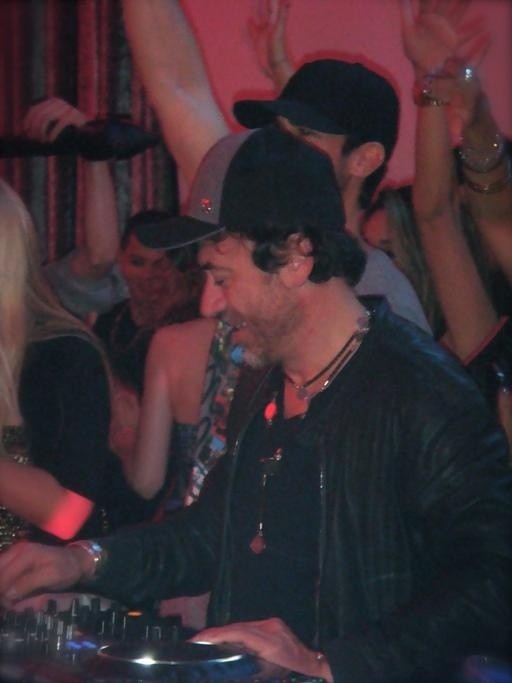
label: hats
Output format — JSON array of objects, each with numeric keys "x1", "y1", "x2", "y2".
[
  {"x1": 232, "y1": 59, "x2": 399, "y2": 161},
  {"x1": 135, "y1": 129, "x2": 346, "y2": 249}
]
